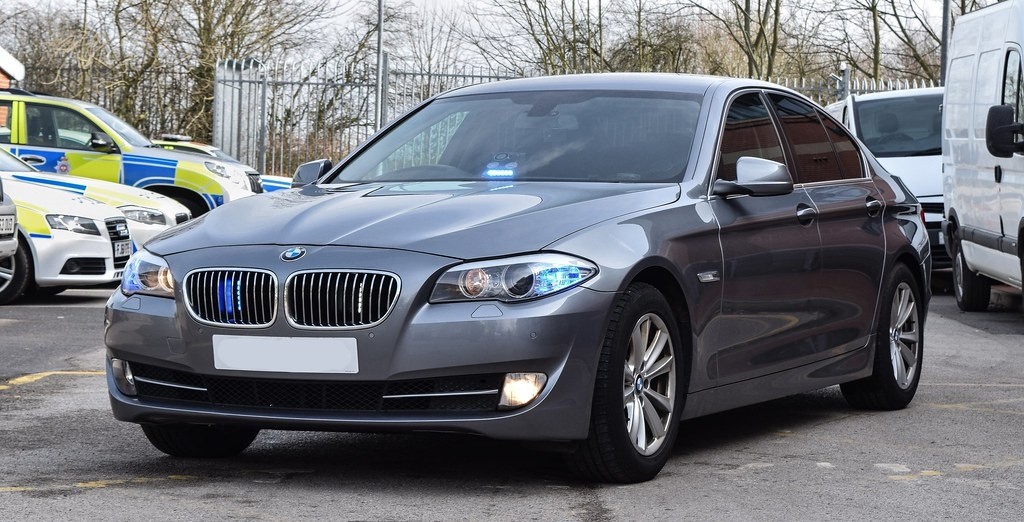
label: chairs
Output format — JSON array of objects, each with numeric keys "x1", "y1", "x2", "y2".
[
  {"x1": 524, "y1": 139, "x2": 579, "y2": 177},
  {"x1": 40, "y1": 118, "x2": 55, "y2": 146},
  {"x1": 28, "y1": 115, "x2": 44, "y2": 145},
  {"x1": 926, "y1": 114, "x2": 942, "y2": 149},
  {"x1": 873, "y1": 111, "x2": 915, "y2": 152}
]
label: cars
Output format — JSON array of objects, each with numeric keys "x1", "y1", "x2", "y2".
[
  {"x1": 0, "y1": 144, "x2": 195, "y2": 255},
  {"x1": 105, "y1": 72, "x2": 934, "y2": 486},
  {"x1": 0, "y1": 127, "x2": 295, "y2": 195},
  {"x1": 1, "y1": 175, "x2": 133, "y2": 306}
]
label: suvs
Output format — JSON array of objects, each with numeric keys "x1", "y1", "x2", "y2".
[{"x1": 0, "y1": 88, "x2": 266, "y2": 238}]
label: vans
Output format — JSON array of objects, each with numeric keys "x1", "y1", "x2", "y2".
[
  {"x1": 943, "y1": 2, "x2": 1024, "y2": 310},
  {"x1": 814, "y1": 87, "x2": 965, "y2": 276}
]
